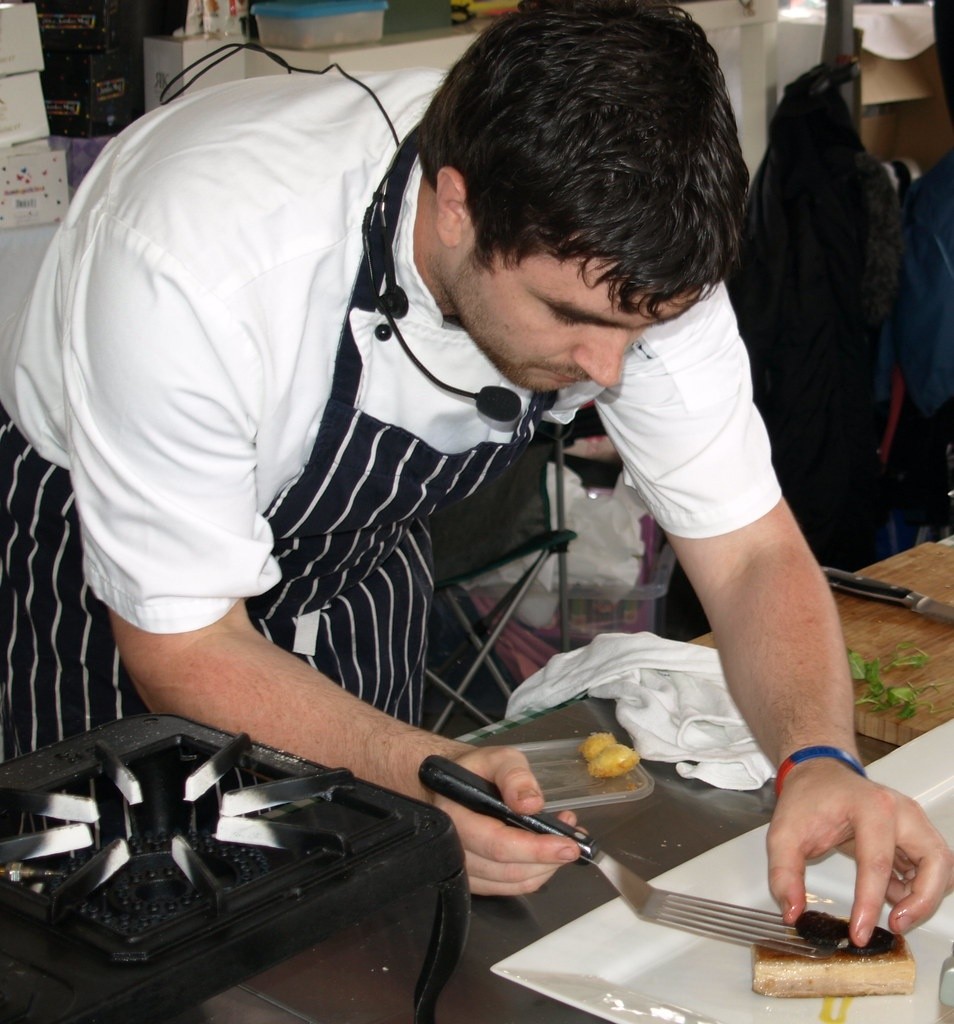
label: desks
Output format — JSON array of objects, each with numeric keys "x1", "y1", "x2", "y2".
[{"x1": 204, "y1": 637, "x2": 905, "y2": 1024}]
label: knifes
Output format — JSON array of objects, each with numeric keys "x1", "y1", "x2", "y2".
[{"x1": 819, "y1": 565, "x2": 954, "y2": 624}]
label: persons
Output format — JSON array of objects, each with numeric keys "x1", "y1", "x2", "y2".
[{"x1": 0, "y1": 1, "x2": 953, "y2": 948}]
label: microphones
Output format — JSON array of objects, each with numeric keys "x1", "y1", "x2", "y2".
[{"x1": 361, "y1": 207, "x2": 522, "y2": 423}]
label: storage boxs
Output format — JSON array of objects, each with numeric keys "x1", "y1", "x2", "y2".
[{"x1": 0, "y1": 0, "x2": 144, "y2": 231}]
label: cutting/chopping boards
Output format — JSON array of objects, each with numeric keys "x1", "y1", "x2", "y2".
[{"x1": 671, "y1": 539, "x2": 954, "y2": 747}]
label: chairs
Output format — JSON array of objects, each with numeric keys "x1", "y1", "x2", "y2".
[{"x1": 419, "y1": 415, "x2": 578, "y2": 737}]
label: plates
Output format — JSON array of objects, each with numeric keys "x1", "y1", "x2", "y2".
[{"x1": 488, "y1": 718, "x2": 952, "y2": 1024}]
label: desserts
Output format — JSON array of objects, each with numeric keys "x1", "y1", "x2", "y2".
[{"x1": 753, "y1": 912, "x2": 915, "y2": 996}]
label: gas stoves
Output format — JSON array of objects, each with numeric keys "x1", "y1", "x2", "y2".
[{"x1": 0, "y1": 713, "x2": 468, "y2": 1024}]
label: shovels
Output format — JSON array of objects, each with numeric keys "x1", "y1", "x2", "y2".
[{"x1": 415, "y1": 753, "x2": 821, "y2": 965}]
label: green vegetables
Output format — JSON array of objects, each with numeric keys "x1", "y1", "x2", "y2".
[{"x1": 846, "y1": 642, "x2": 954, "y2": 718}]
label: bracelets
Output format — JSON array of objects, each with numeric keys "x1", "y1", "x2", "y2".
[{"x1": 773, "y1": 745, "x2": 867, "y2": 797}]
